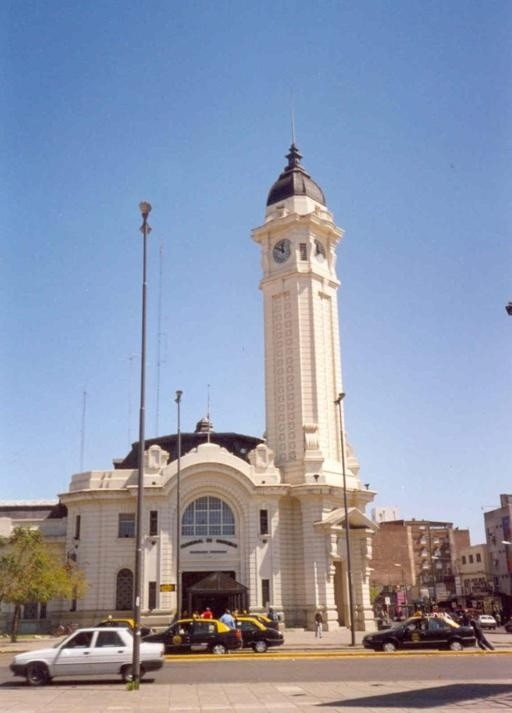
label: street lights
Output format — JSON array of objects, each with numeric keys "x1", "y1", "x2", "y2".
[
  {"x1": 333, "y1": 393, "x2": 356, "y2": 645},
  {"x1": 173, "y1": 389, "x2": 183, "y2": 619},
  {"x1": 500, "y1": 538, "x2": 512, "y2": 546},
  {"x1": 387, "y1": 560, "x2": 401, "y2": 591},
  {"x1": 129, "y1": 198, "x2": 151, "y2": 686},
  {"x1": 430, "y1": 555, "x2": 455, "y2": 561}
]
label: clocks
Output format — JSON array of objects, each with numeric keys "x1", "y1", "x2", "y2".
[
  {"x1": 272, "y1": 239, "x2": 291, "y2": 263},
  {"x1": 314, "y1": 239, "x2": 325, "y2": 262}
]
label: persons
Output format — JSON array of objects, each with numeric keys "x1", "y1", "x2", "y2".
[
  {"x1": 459, "y1": 609, "x2": 496, "y2": 651},
  {"x1": 314, "y1": 611, "x2": 325, "y2": 639},
  {"x1": 267, "y1": 608, "x2": 273, "y2": 620},
  {"x1": 182, "y1": 607, "x2": 213, "y2": 619},
  {"x1": 218, "y1": 608, "x2": 235, "y2": 629},
  {"x1": 414, "y1": 608, "x2": 422, "y2": 617}
]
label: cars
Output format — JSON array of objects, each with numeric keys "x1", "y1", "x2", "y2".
[
  {"x1": 362, "y1": 610, "x2": 498, "y2": 654},
  {"x1": 10, "y1": 612, "x2": 285, "y2": 688}
]
